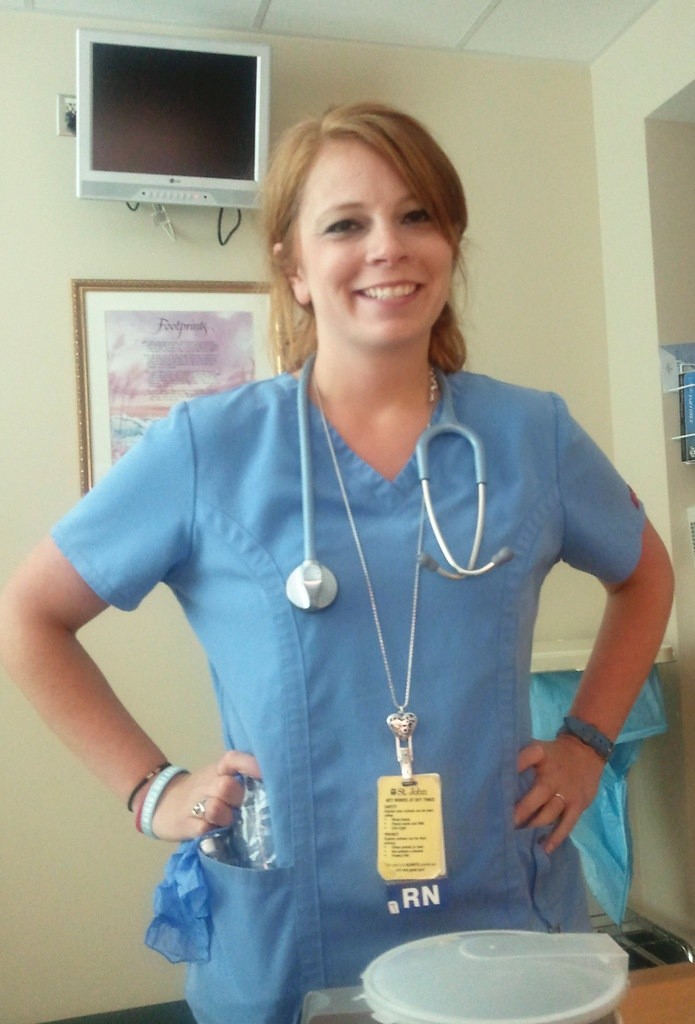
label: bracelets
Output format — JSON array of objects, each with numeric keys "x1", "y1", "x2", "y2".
[
  {"x1": 556, "y1": 715, "x2": 616, "y2": 762},
  {"x1": 135, "y1": 763, "x2": 192, "y2": 842}
]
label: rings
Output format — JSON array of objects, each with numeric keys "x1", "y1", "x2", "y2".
[
  {"x1": 189, "y1": 796, "x2": 212, "y2": 821},
  {"x1": 553, "y1": 793, "x2": 570, "y2": 808}
]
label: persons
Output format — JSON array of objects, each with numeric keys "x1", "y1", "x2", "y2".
[{"x1": 4, "y1": 101, "x2": 677, "y2": 1009}]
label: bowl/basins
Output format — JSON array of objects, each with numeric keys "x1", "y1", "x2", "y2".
[{"x1": 358, "y1": 929, "x2": 629, "y2": 1023}]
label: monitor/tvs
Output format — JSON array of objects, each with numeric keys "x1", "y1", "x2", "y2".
[{"x1": 73, "y1": 28, "x2": 271, "y2": 209}]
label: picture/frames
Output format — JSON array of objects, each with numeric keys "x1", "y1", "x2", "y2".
[{"x1": 73, "y1": 278, "x2": 283, "y2": 511}]
